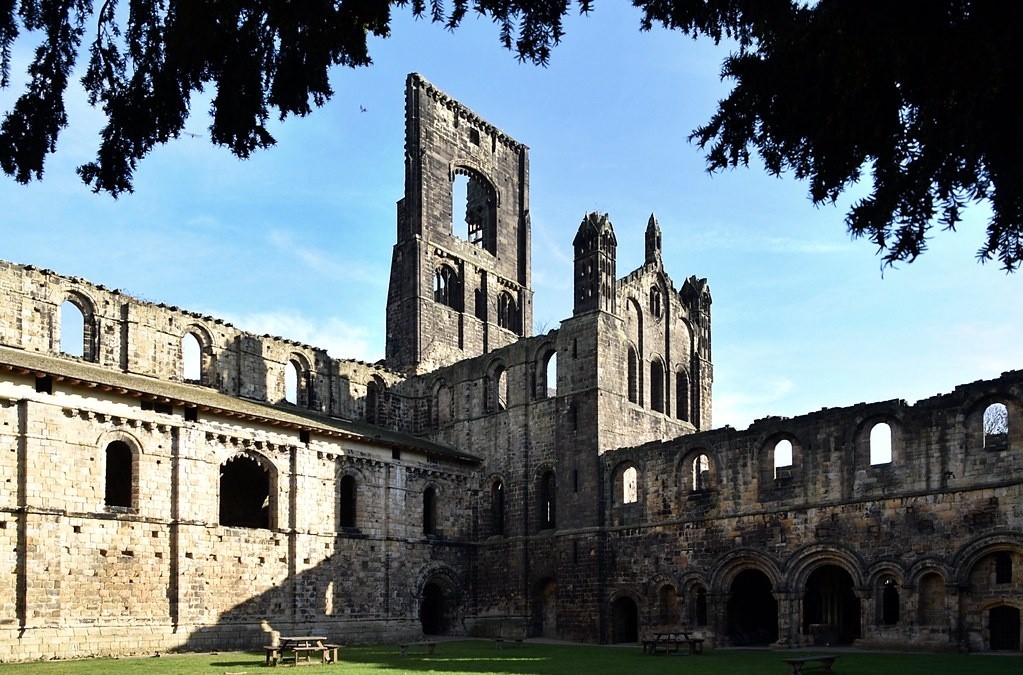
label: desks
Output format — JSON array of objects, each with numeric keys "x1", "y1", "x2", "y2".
[
  {"x1": 277, "y1": 636, "x2": 332, "y2": 663},
  {"x1": 649, "y1": 631, "x2": 696, "y2": 656}
]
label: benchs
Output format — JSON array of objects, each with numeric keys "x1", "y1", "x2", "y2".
[
  {"x1": 779, "y1": 653, "x2": 842, "y2": 675},
  {"x1": 639, "y1": 638, "x2": 706, "y2": 645},
  {"x1": 495, "y1": 636, "x2": 528, "y2": 650},
  {"x1": 280, "y1": 643, "x2": 312, "y2": 662},
  {"x1": 293, "y1": 645, "x2": 329, "y2": 667},
  {"x1": 397, "y1": 639, "x2": 438, "y2": 657},
  {"x1": 323, "y1": 640, "x2": 343, "y2": 661},
  {"x1": 262, "y1": 644, "x2": 281, "y2": 666}
]
